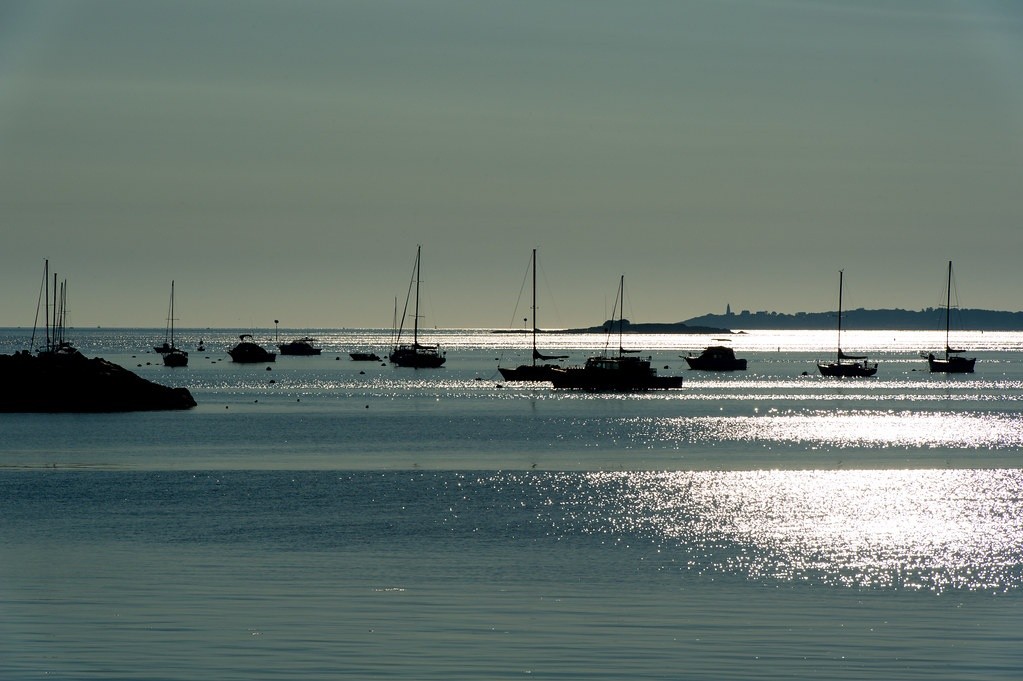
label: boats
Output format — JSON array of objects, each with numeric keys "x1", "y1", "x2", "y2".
[
  {"x1": 228, "y1": 334, "x2": 276, "y2": 363},
  {"x1": 348, "y1": 353, "x2": 380, "y2": 361},
  {"x1": 679, "y1": 346, "x2": 748, "y2": 371},
  {"x1": 544, "y1": 275, "x2": 683, "y2": 390},
  {"x1": 275, "y1": 336, "x2": 322, "y2": 355}
]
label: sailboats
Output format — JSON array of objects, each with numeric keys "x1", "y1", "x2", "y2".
[
  {"x1": 928, "y1": 260, "x2": 977, "y2": 373},
  {"x1": 25, "y1": 258, "x2": 79, "y2": 357},
  {"x1": 150, "y1": 278, "x2": 189, "y2": 367},
  {"x1": 389, "y1": 244, "x2": 447, "y2": 368},
  {"x1": 496, "y1": 247, "x2": 563, "y2": 380},
  {"x1": 816, "y1": 269, "x2": 879, "y2": 378}
]
